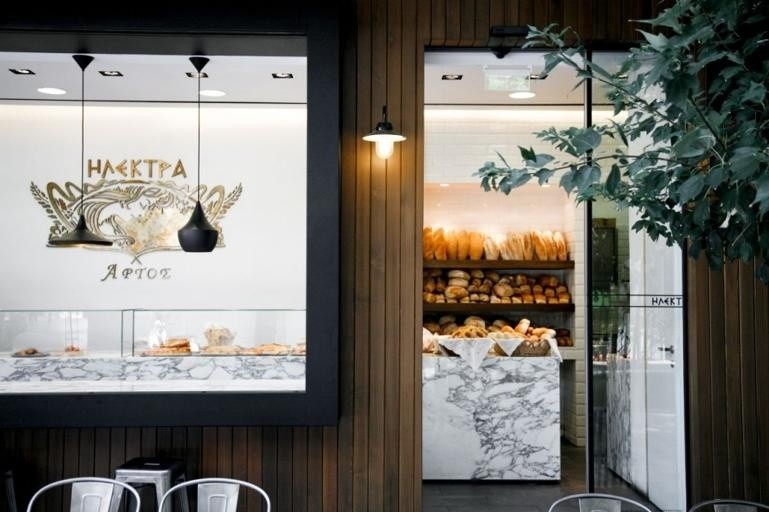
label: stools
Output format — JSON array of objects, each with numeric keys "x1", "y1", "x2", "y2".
[{"x1": 108, "y1": 457, "x2": 191, "y2": 511}]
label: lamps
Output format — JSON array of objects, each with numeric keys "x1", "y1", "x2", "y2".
[
  {"x1": 46, "y1": 56, "x2": 115, "y2": 250},
  {"x1": 179, "y1": 56, "x2": 220, "y2": 252},
  {"x1": 362, "y1": 2, "x2": 409, "y2": 159}
]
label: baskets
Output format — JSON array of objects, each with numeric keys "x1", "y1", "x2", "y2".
[{"x1": 493, "y1": 340, "x2": 550, "y2": 357}]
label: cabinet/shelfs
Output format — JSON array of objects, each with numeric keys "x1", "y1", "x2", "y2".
[{"x1": 423, "y1": 259, "x2": 574, "y2": 365}]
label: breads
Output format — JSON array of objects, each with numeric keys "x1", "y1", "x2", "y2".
[
  {"x1": 144, "y1": 326, "x2": 306, "y2": 355},
  {"x1": 422, "y1": 225, "x2": 573, "y2": 355}
]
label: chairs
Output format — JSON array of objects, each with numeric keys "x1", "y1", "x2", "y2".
[
  {"x1": 26, "y1": 474, "x2": 273, "y2": 512},
  {"x1": 548, "y1": 491, "x2": 769, "y2": 512}
]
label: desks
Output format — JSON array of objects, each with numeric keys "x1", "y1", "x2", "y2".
[{"x1": 422, "y1": 355, "x2": 564, "y2": 484}]
